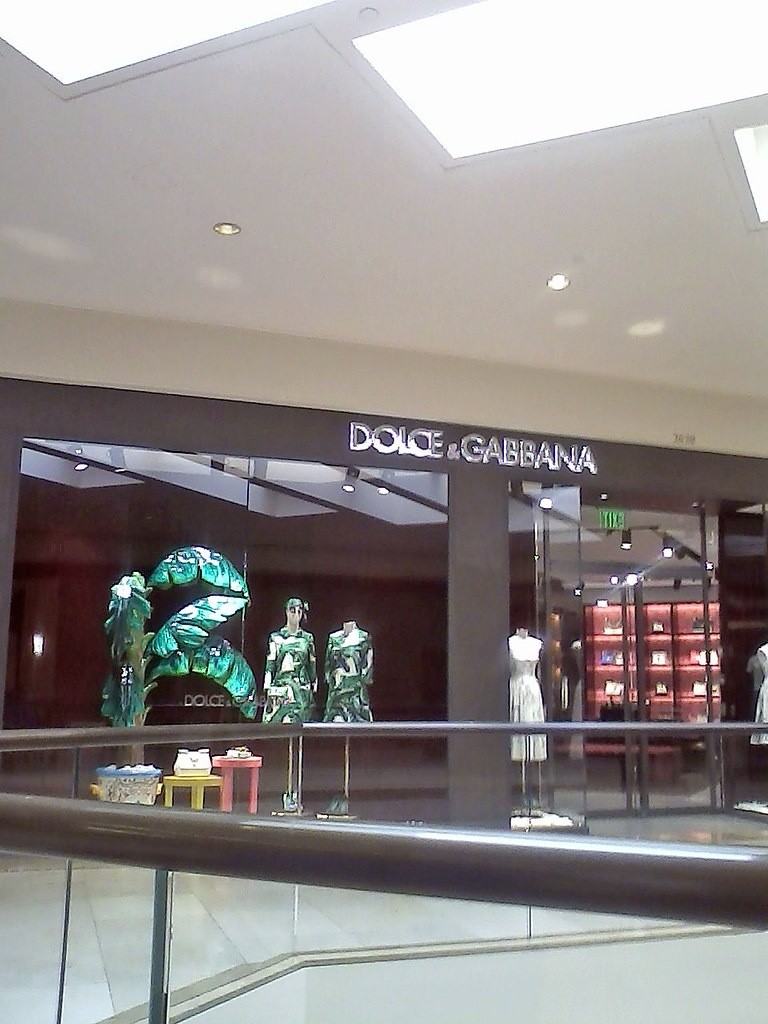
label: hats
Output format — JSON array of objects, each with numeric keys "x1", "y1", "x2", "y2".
[{"x1": 287, "y1": 598, "x2": 303, "y2": 607}]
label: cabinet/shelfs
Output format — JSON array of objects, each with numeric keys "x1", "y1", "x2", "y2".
[{"x1": 583, "y1": 600, "x2": 723, "y2": 754}]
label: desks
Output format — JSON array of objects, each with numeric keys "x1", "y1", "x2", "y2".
[{"x1": 163, "y1": 773, "x2": 221, "y2": 808}]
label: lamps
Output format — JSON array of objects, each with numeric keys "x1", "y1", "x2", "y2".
[
  {"x1": 107, "y1": 448, "x2": 129, "y2": 474},
  {"x1": 68, "y1": 445, "x2": 89, "y2": 472},
  {"x1": 342, "y1": 474, "x2": 357, "y2": 494},
  {"x1": 620, "y1": 529, "x2": 633, "y2": 552}
]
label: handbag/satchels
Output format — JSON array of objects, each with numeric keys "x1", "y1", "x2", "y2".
[
  {"x1": 598, "y1": 614, "x2": 722, "y2": 698},
  {"x1": 173, "y1": 748, "x2": 212, "y2": 778}
]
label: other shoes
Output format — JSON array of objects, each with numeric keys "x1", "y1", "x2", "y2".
[
  {"x1": 284, "y1": 792, "x2": 302, "y2": 811},
  {"x1": 325, "y1": 793, "x2": 348, "y2": 815}
]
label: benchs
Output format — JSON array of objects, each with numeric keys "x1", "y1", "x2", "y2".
[
  {"x1": 213, "y1": 752, "x2": 263, "y2": 815},
  {"x1": 523, "y1": 743, "x2": 680, "y2": 789}
]
